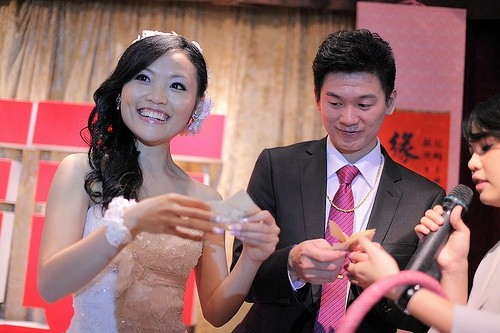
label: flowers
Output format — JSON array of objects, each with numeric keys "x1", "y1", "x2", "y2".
[{"x1": 181, "y1": 92, "x2": 213, "y2": 137}]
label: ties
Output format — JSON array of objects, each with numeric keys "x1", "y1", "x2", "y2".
[{"x1": 312, "y1": 165, "x2": 360, "y2": 333}]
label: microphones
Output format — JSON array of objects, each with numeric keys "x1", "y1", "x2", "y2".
[{"x1": 404, "y1": 184, "x2": 474, "y2": 272}]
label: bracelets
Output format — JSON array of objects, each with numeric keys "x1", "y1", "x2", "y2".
[
  {"x1": 394, "y1": 285, "x2": 421, "y2": 316},
  {"x1": 101, "y1": 192, "x2": 140, "y2": 249}
]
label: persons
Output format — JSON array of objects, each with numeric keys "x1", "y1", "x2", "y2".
[
  {"x1": 230, "y1": 28, "x2": 447, "y2": 333},
  {"x1": 36, "y1": 30, "x2": 281, "y2": 333},
  {"x1": 344, "y1": 93, "x2": 500, "y2": 333}
]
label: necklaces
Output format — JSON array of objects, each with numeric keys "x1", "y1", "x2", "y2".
[{"x1": 326, "y1": 155, "x2": 383, "y2": 213}]
